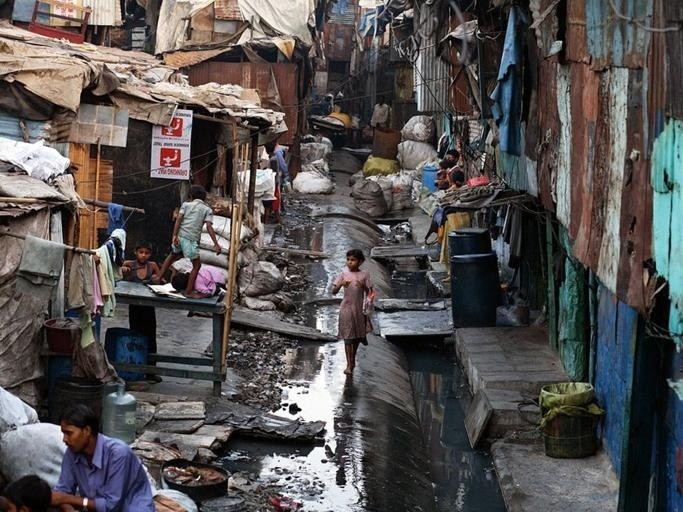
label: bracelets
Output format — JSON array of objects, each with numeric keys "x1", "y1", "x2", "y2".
[{"x1": 83, "y1": 497, "x2": 88, "y2": 507}]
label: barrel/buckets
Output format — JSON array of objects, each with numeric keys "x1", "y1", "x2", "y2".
[
  {"x1": 449, "y1": 253, "x2": 499, "y2": 328},
  {"x1": 44, "y1": 315, "x2": 83, "y2": 348},
  {"x1": 372, "y1": 127, "x2": 402, "y2": 161},
  {"x1": 422, "y1": 166, "x2": 440, "y2": 194},
  {"x1": 47, "y1": 357, "x2": 74, "y2": 380},
  {"x1": 448, "y1": 229, "x2": 492, "y2": 257},
  {"x1": 103, "y1": 384, "x2": 138, "y2": 445},
  {"x1": 46, "y1": 377, "x2": 104, "y2": 432},
  {"x1": 105, "y1": 326, "x2": 148, "y2": 381}
]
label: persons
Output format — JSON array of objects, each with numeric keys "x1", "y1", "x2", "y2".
[
  {"x1": 332, "y1": 250, "x2": 376, "y2": 374},
  {"x1": 260, "y1": 143, "x2": 289, "y2": 224},
  {"x1": 152, "y1": 184, "x2": 226, "y2": 302},
  {"x1": 1, "y1": 474, "x2": 51, "y2": 512},
  {"x1": 121, "y1": 240, "x2": 165, "y2": 382},
  {"x1": 51, "y1": 404, "x2": 154, "y2": 512},
  {"x1": 310, "y1": 89, "x2": 345, "y2": 116},
  {"x1": 433, "y1": 149, "x2": 473, "y2": 282},
  {"x1": 368, "y1": 96, "x2": 389, "y2": 148}
]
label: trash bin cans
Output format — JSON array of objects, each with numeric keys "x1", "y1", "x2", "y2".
[
  {"x1": 448, "y1": 227, "x2": 503, "y2": 328},
  {"x1": 55, "y1": 377, "x2": 104, "y2": 435}
]
